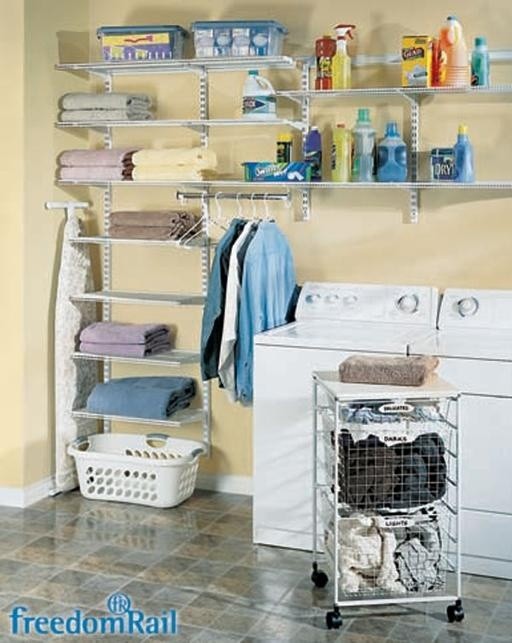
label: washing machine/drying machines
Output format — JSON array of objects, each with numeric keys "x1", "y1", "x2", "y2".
[
  {"x1": 408, "y1": 287, "x2": 512, "y2": 579},
  {"x1": 252, "y1": 281, "x2": 441, "y2": 552}
]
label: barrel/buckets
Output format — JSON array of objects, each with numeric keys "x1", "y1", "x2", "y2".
[
  {"x1": 377, "y1": 122, "x2": 408, "y2": 182},
  {"x1": 242, "y1": 70, "x2": 277, "y2": 122},
  {"x1": 436, "y1": 15, "x2": 469, "y2": 87}
]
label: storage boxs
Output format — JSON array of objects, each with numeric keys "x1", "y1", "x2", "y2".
[{"x1": 98, "y1": 20, "x2": 287, "y2": 63}]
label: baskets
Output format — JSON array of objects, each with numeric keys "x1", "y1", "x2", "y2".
[{"x1": 67, "y1": 434, "x2": 206, "y2": 509}]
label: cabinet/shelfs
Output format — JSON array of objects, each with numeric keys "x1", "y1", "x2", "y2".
[{"x1": 47, "y1": 51, "x2": 512, "y2": 454}]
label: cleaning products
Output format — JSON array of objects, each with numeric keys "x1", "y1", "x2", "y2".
[
  {"x1": 352, "y1": 109, "x2": 378, "y2": 181},
  {"x1": 332, "y1": 22, "x2": 356, "y2": 89},
  {"x1": 330, "y1": 123, "x2": 350, "y2": 180},
  {"x1": 470, "y1": 37, "x2": 490, "y2": 83},
  {"x1": 453, "y1": 124, "x2": 474, "y2": 181}
]
